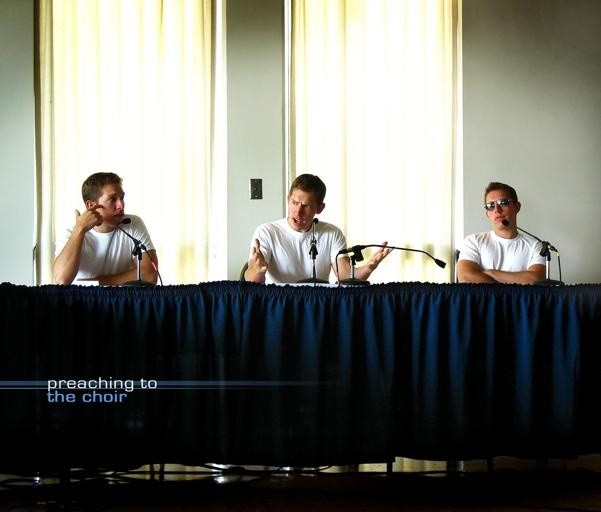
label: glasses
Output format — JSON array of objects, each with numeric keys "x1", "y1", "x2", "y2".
[{"x1": 484, "y1": 198, "x2": 512, "y2": 211}]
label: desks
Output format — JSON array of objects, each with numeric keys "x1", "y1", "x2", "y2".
[{"x1": 0, "y1": 279, "x2": 600, "y2": 478}]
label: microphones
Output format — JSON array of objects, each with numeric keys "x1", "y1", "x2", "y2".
[
  {"x1": 115, "y1": 216, "x2": 155, "y2": 287},
  {"x1": 336, "y1": 242, "x2": 449, "y2": 284},
  {"x1": 296, "y1": 217, "x2": 329, "y2": 283},
  {"x1": 501, "y1": 217, "x2": 566, "y2": 286}
]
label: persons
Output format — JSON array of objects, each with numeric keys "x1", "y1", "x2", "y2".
[
  {"x1": 241, "y1": 174, "x2": 395, "y2": 289},
  {"x1": 49, "y1": 171, "x2": 159, "y2": 288},
  {"x1": 458, "y1": 179, "x2": 548, "y2": 288}
]
